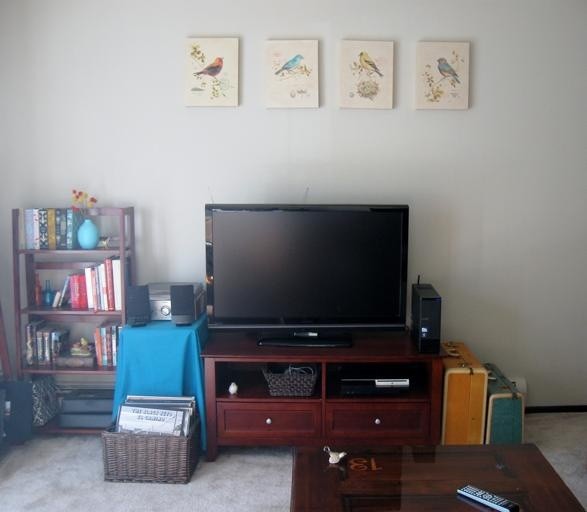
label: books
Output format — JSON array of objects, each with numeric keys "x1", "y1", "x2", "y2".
[
  {"x1": 117, "y1": 395, "x2": 196, "y2": 437},
  {"x1": 24, "y1": 208, "x2": 121, "y2": 370}
]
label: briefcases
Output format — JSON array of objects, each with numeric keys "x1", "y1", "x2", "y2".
[
  {"x1": 441, "y1": 341, "x2": 487, "y2": 445},
  {"x1": 484, "y1": 363, "x2": 525, "y2": 444}
]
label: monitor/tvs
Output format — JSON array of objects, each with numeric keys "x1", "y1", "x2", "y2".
[{"x1": 205, "y1": 204, "x2": 409, "y2": 348}]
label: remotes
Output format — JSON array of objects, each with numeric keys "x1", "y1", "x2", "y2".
[{"x1": 457, "y1": 484, "x2": 520, "y2": 512}]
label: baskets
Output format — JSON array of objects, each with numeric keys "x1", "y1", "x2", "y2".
[
  {"x1": 102, "y1": 423, "x2": 201, "y2": 484},
  {"x1": 264, "y1": 366, "x2": 316, "y2": 397}
]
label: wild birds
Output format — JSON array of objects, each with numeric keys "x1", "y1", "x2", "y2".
[
  {"x1": 275, "y1": 54, "x2": 305, "y2": 75},
  {"x1": 436, "y1": 58, "x2": 461, "y2": 84},
  {"x1": 195, "y1": 56, "x2": 226, "y2": 79},
  {"x1": 357, "y1": 51, "x2": 384, "y2": 78}
]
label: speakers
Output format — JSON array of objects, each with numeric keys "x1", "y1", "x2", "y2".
[
  {"x1": 170, "y1": 285, "x2": 194, "y2": 324},
  {"x1": 127, "y1": 285, "x2": 150, "y2": 326}
]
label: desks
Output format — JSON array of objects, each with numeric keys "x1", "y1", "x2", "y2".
[
  {"x1": 290, "y1": 443, "x2": 587, "y2": 512},
  {"x1": 112, "y1": 311, "x2": 207, "y2": 452}
]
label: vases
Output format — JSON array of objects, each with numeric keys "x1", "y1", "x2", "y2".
[{"x1": 77, "y1": 218, "x2": 101, "y2": 250}]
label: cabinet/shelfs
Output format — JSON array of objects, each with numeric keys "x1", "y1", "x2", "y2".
[
  {"x1": 200, "y1": 329, "x2": 442, "y2": 462},
  {"x1": 11, "y1": 206, "x2": 136, "y2": 434}
]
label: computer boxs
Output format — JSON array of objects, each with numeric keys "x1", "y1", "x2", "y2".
[{"x1": 412, "y1": 283, "x2": 441, "y2": 354}]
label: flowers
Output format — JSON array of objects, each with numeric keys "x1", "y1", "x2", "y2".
[{"x1": 70, "y1": 189, "x2": 97, "y2": 220}]
